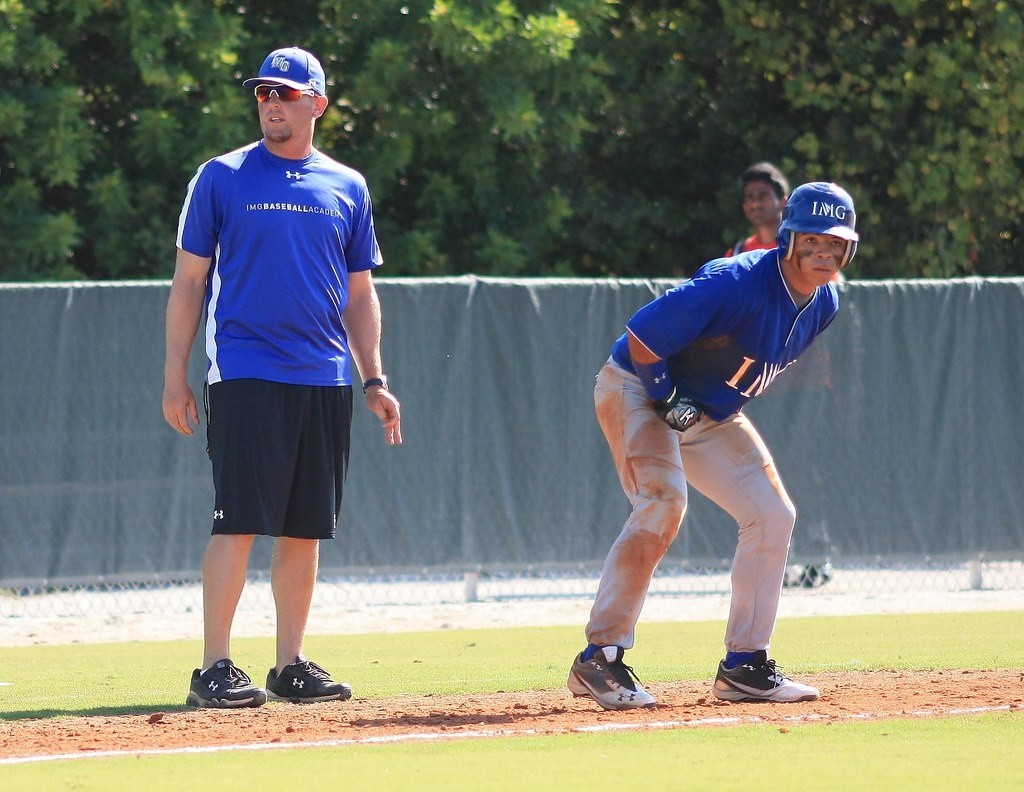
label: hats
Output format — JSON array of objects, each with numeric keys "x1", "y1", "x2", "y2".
[{"x1": 242, "y1": 47, "x2": 326, "y2": 96}]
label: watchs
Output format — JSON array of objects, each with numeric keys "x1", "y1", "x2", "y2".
[{"x1": 362, "y1": 376, "x2": 389, "y2": 393}]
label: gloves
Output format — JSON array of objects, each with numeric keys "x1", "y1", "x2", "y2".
[{"x1": 653, "y1": 385, "x2": 705, "y2": 432}]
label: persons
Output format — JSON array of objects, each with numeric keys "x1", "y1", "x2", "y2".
[
  {"x1": 723, "y1": 162, "x2": 861, "y2": 591},
  {"x1": 162, "y1": 45, "x2": 401, "y2": 706},
  {"x1": 568, "y1": 181, "x2": 860, "y2": 710}
]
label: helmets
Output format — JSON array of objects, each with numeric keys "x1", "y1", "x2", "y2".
[{"x1": 776, "y1": 182, "x2": 861, "y2": 266}]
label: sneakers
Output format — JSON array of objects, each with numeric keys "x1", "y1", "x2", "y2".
[
  {"x1": 185, "y1": 658, "x2": 268, "y2": 710},
  {"x1": 265, "y1": 654, "x2": 352, "y2": 704},
  {"x1": 566, "y1": 645, "x2": 657, "y2": 711},
  {"x1": 711, "y1": 657, "x2": 820, "y2": 704}
]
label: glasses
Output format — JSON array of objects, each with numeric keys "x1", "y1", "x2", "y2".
[{"x1": 253, "y1": 83, "x2": 322, "y2": 102}]
